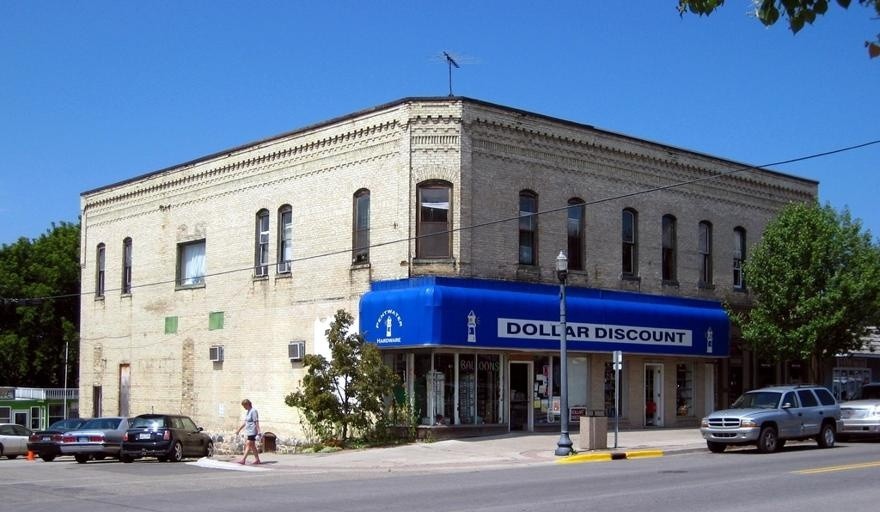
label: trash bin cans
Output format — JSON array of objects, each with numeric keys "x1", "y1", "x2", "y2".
[
  {"x1": 262, "y1": 432, "x2": 277, "y2": 453},
  {"x1": 579, "y1": 408, "x2": 608, "y2": 450}
]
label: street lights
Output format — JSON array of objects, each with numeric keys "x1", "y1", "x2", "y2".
[{"x1": 554, "y1": 245, "x2": 575, "y2": 456}]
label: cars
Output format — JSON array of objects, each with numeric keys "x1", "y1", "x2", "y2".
[{"x1": 0, "y1": 413, "x2": 216, "y2": 465}]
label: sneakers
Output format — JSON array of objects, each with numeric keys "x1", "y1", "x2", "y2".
[
  {"x1": 235, "y1": 461, "x2": 246, "y2": 465},
  {"x1": 251, "y1": 460, "x2": 261, "y2": 465}
]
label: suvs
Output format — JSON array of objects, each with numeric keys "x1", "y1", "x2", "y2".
[
  {"x1": 700, "y1": 382, "x2": 846, "y2": 454},
  {"x1": 839, "y1": 381, "x2": 880, "y2": 441}
]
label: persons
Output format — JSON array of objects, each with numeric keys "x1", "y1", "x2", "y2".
[{"x1": 233, "y1": 399, "x2": 264, "y2": 465}]
